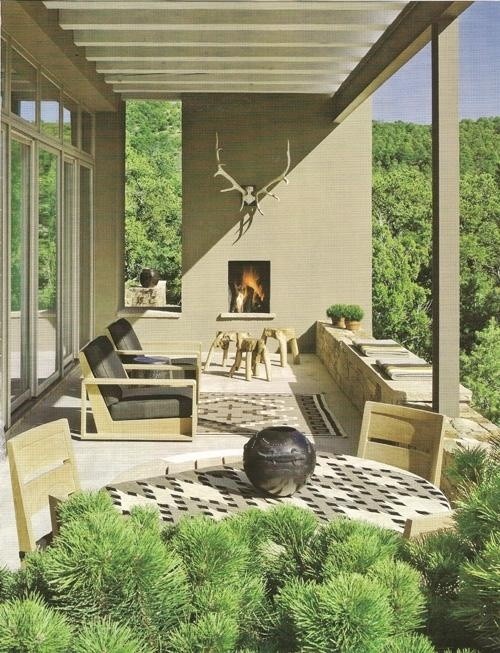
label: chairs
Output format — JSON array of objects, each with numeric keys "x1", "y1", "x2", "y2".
[
  {"x1": 357, "y1": 399, "x2": 449, "y2": 490},
  {"x1": 79, "y1": 334, "x2": 199, "y2": 444},
  {"x1": 102, "y1": 316, "x2": 202, "y2": 404},
  {"x1": 403, "y1": 519, "x2": 451, "y2": 539},
  {"x1": 6, "y1": 418, "x2": 83, "y2": 566}
]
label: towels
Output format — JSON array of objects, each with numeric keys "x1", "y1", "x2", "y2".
[{"x1": 197, "y1": 392, "x2": 348, "y2": 439}]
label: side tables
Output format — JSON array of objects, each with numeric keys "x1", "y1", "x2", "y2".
[{"x1": 132, "y1": 358, "x2": 169, "y2": 378}]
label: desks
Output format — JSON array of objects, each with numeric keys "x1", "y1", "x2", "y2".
[{"x1": 99, "y1": 450, "x2": 450, "y2": 542}]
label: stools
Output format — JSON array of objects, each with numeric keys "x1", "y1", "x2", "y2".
[
  {"x1": 261, "y1": 327, "x2": 301, "y2": 367},
  {"x1": 229, "y1": 338, "x2": 271, "y2": 382},
  {"x1": 204, "y1": 330, "x2": 252, "y2": 371}
]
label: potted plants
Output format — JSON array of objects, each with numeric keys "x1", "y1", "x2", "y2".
[
  {"x1": 326, "y1": 303, "x2": 345, "y2": 328},
  {"x1": 344, "y1": 304, "x2": 364, "y2": 330}
]
